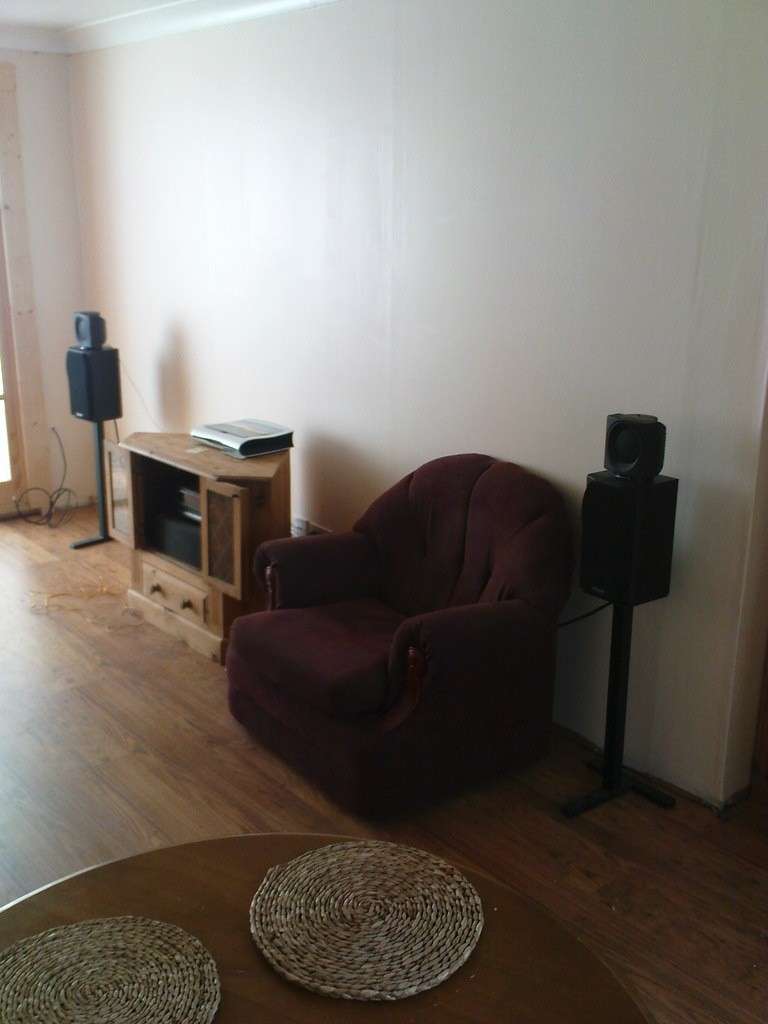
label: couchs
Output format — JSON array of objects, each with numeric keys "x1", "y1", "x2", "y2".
[{"x1": 227, "y1": 453, "x2": 571, "y2": 824}]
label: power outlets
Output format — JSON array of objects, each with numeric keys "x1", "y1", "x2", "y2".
[{"x1": 291, "y1": 517, "x2": 334, "y2": 537}]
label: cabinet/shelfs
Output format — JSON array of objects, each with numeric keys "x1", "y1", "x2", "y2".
[{"x1": 104, "y1": 432, "x2": 292, "y2": 665}]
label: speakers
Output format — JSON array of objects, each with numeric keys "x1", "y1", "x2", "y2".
[
  {"x1": 74, "y1": 312, "x2": 106, "y2": 350},
  {"x1": 604, "y1": 413, "x2": 665, "y2": 482},
  {"x1": 577, "y1": 470, "x2": 678, "y2": 607},
  {"x1": 67, "y1": 345, "x2": 123, "y2": 422}
]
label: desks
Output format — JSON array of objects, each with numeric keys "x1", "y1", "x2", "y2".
[{"x1": 0, "y1": 834, "x2": 646, "y2": 1024}]
